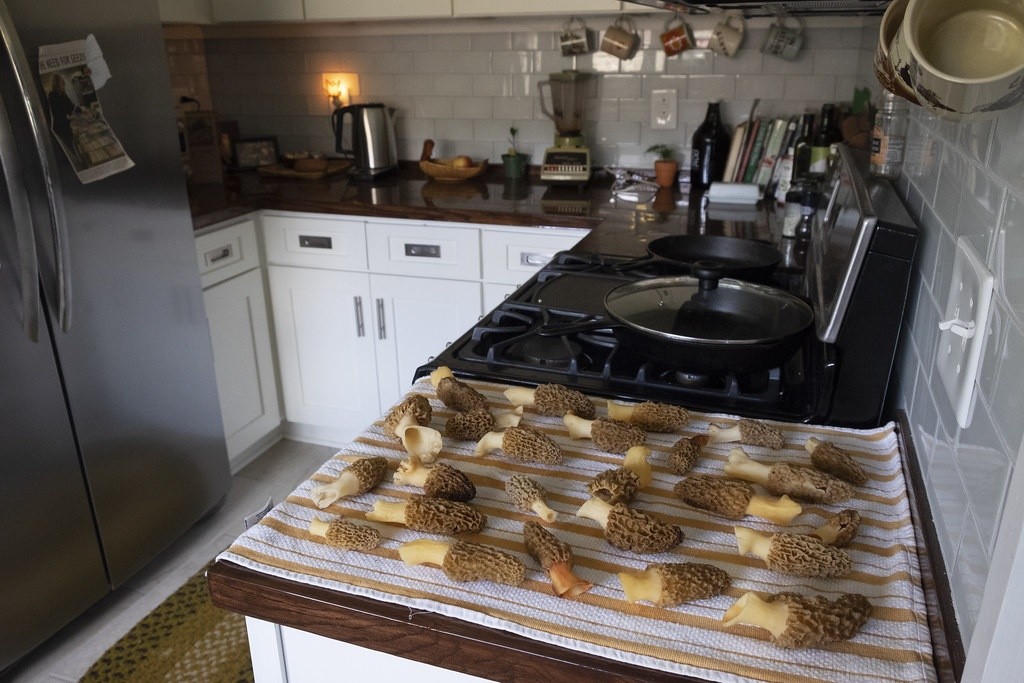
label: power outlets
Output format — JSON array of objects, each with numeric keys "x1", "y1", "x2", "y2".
[{"x1": 175, "y1": 87, "x2": 194, "y2": 112}]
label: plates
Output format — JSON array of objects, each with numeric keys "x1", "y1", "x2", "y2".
[{"x1": 420, "y1": 158, "x2": 487, "y2": 181}]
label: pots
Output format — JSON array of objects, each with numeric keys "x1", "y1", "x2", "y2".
[
  {"x1": 612, "y1": 234, "x2": 781, "y2": 285},
  {"x1": 540, "y1": 262, "x2": 815, "y2": 373}
]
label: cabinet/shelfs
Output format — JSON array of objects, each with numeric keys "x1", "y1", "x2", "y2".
[
  {"x1": 198, "y1": 218, "x2": 284, "y2": 476},
  {"x1": 257, "y1": 213, "x2": 482, "y2": 450},
  {"x1": 480, "y1": 229, "x2": 586, "y2": 322}
]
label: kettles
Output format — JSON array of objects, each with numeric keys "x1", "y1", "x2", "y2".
[{"x1": 330, "y1": 103, "x2": 398, "y2": 177}]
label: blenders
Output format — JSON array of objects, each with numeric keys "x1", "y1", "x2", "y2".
[{"x1": 537, "y1": 68, "x2": 591, "y2": 195}]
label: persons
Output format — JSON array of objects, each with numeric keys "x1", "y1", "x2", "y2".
[{"x1": 49, "y1": 74, "x2": 99, "y2": 164}]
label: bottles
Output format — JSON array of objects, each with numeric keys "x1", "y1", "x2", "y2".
[{"x1": 679, "y1": 85, "x2": 908, "y2": 239}]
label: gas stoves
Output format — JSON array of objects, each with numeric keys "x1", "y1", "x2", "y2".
[{"x1": 413, "y1": 142, "x2": 918, "y2": 428}]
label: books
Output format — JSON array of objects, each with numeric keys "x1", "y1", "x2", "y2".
[{"x1": 722, "y1": 107, "x2": 819, "y2": 197}]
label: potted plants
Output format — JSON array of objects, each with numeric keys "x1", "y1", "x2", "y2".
[
  {"x1": 647, "y1": 143, "x2": 680, "y2": 188},
  {"x1": 500, "y1": 126, "x2": 529, "y2": 178}
]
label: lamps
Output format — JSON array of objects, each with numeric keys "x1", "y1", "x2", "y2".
[{"x1": 323, "y1": 79, "x2": 345, "y2": 111}]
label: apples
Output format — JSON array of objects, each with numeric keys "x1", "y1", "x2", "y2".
[{"x1": 453, "y1": 155, "x2": 472, "y2": 168}]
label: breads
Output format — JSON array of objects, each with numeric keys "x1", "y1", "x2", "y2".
[{"x1": 294, "y1": 158, "x2": 328, "y2": 171}]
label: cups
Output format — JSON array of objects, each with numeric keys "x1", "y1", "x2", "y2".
[
  {"x1": 872, "y1": 0, "x2": 1023, "y2": 122},
  {"x1": 600, "y1": 16, "x2": 638, "y2": 60},
  {"x1": 760, "y1": 14, "x2": 804, "y2": 60},
  {"x1": 707, "y1": 14, "x2": 746, "y2": 57},
  {"x1": 559, "y1": 18, "x2": 589, "y2": 56},
  {"x1": 660, "y1": 16, "x2": 695, "y2": 57}
]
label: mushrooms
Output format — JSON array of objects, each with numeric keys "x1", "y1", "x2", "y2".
[{"x1": 310, "y1": 364, "x2": 872, "y2": 649}]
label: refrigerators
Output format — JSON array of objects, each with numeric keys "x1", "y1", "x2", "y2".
[{"x1": 0, "y1": 0, "x2": 232, "y2": 683}]
